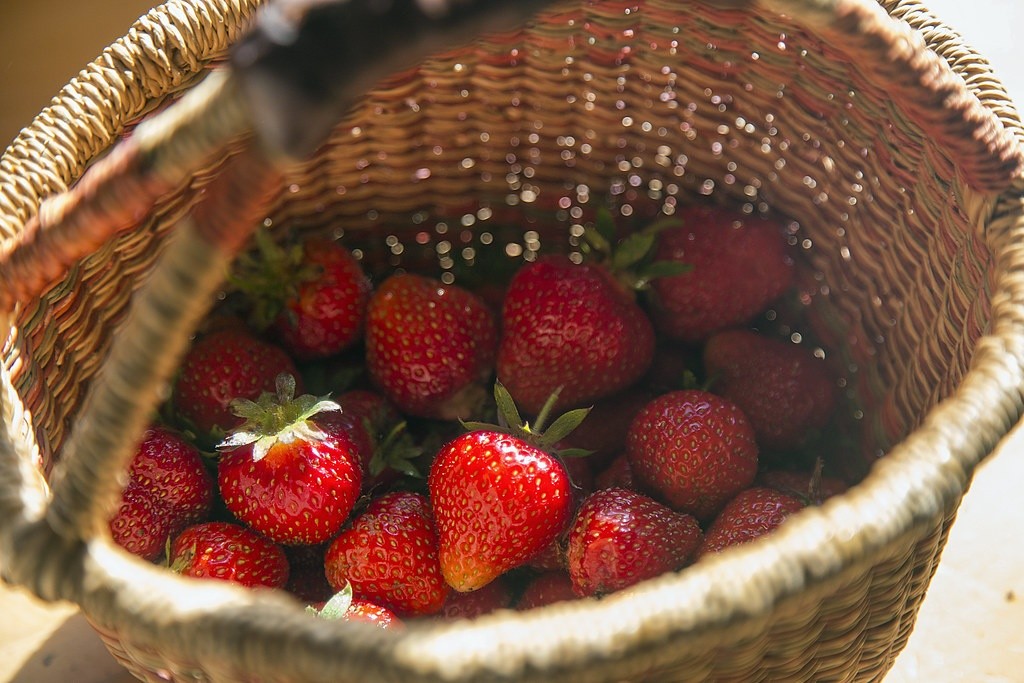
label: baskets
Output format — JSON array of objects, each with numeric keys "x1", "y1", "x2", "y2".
[{"x1": 0, "y1": 1, "x2": 1024, "y2": 682}]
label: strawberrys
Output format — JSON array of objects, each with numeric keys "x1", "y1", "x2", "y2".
[{"x1": 113, "y1": 201, "x2": 849, "y2": 625}]
